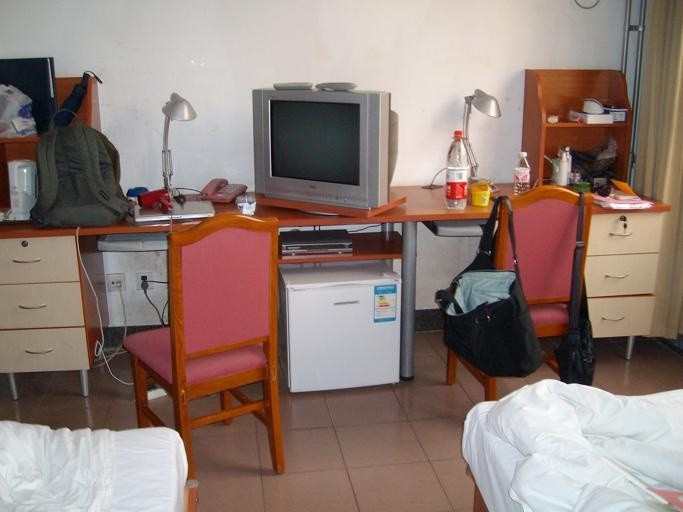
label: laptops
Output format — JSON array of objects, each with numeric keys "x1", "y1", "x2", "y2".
[{"x1": 133, "y1": 200, "x2": 215, "y2": 222}]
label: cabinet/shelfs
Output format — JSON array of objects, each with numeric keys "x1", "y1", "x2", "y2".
[
  {"x1": 0, "y1": 77, "x2": 101, "y2": 212},
  {"x1": 520, "y1": 69, "x2": 633, "y2": 191}
]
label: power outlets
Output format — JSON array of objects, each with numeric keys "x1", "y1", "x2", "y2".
[
  {"x1": 136, "y1": 272, "x2": 154, "y2": 290},
  {"x1": 107, "y1": 273, "x2": 126, "y2": 292}
]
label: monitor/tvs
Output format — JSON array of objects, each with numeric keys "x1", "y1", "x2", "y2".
[{"x1": 252, "y1": 89, "x2": 398, "y2": 212}]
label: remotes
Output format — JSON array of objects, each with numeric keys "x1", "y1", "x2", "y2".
[
  {"x1": 317, "y1": 82, "x2": 357, "y2": 92},
  {"x1": 273, "y1": 82, "x2": 313, "y2": 91}
]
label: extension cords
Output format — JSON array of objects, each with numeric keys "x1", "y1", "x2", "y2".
[{"x1": 146, "y1": 386, "x2": 169, "y2": 400}]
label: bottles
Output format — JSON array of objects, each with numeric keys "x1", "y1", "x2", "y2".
[
  {"x1": 514, "y1": 151, "x2": 530, "y2": 197},
  {"x1": 468, "y1": 175, "x2": 492, "y2": 207},
  {"x1": 560, "y1": 145, "x2": 572, "y2": 184},
  {"x1": 568, "y1": 173, "x2": 580, "y2": 184},
  {"x1": 443, "y1": 130, "x2": 470, "y2": 212}
]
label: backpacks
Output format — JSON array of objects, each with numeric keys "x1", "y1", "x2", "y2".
[{"x1": 30, "y1": 110, "x2": 128, "y2": 227}]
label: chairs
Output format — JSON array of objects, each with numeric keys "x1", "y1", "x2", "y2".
[
  {"x1": 434, "y1": 185, "x2": 593, "y2": 402},
  {"x1": 123, "y1": 212, "x2": 285, "y2": 479}
]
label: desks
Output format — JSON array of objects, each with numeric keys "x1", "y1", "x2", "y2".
[{"x1": 0, "y1": 183, "x2": 670, "y2": 398}]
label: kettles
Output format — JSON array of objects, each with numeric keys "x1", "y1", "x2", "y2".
[{"x1": 6, "y1": 158, "x2": 40, "y2": 221}]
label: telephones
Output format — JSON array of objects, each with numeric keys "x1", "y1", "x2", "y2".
[{"x1": 199, "y1": 178, "x2": 247, "y2": 202}]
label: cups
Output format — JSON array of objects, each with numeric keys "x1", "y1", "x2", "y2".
[{"x1": 233, "y1": 194, "x2": 256, "y2": 217}]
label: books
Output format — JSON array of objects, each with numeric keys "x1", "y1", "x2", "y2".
[{"x1": 593, "y1": 178, "x2": 653, "y2": 210}]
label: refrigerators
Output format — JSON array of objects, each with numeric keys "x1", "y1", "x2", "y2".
[{"x1": 279, "y1": 262, "x2": 400, "y2": 394}]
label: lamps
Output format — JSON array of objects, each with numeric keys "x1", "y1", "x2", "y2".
[
  {"x1": 161, "y1": 93, "x2": 197, "y2": 188},
  {"x1": 462, "y1": 89, "x2": 501, "y2": 176}
]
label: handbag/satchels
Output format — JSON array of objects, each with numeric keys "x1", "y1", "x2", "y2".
[
  {"x1": 436, "y1": 254, "x2": 542, "y2": 378},
  {"x1": 557, "y1": 316, "x2": 595, "y2": 386}
]
